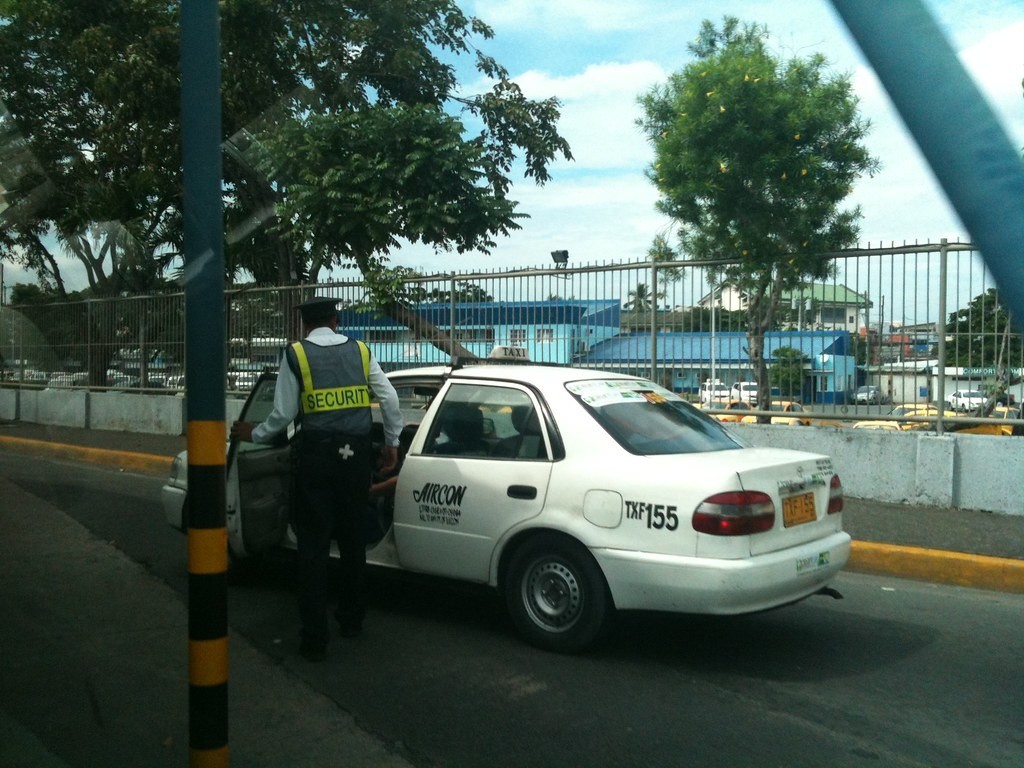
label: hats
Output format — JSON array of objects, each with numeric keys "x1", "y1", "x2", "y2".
[{"x1": 293, "y1": 296, "x2": 343, "y2": 314}]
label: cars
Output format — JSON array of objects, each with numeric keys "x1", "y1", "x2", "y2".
[
  {"x1": 161, "y1": 343, "x2": 851, "y2": 656},
  {"x1": 0, "y1": 338, "x2": 281, "y2": 397},
  {"x1": 689, "y1": 396, "x2": 1023, "y2": 438},
  {"x1": 948, "y1": 390, "x2": 988, "y2": 412},
  {"x1": 851, "y1": 384, "x2": 888, "y2": 407}
]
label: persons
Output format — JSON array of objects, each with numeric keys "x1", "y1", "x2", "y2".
[{"x1": 231, "y1": 299, "x2": 401, "y2": 661}]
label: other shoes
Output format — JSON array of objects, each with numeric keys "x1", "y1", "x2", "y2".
[
  {"x1": 299, "y1": 640, "x2": 328, "y2": 662},
  {"x1": 340, "y1": 622, "x2": 363, "y2": 638}
]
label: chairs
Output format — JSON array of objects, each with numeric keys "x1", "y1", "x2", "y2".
[
  {"x1": 490, "y1": 401, "x2": 543, "y2": 458},
  {"x1": 435, "y1": 407, "x2": 494, "y2": 457}
]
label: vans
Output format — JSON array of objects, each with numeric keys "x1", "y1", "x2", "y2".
[
  {"x1": 698, "y1": 379, "x2": 730, "y2": 404},
  {"x1": 732, "y1": 381, "x2": 759, "y2": 407}
]
